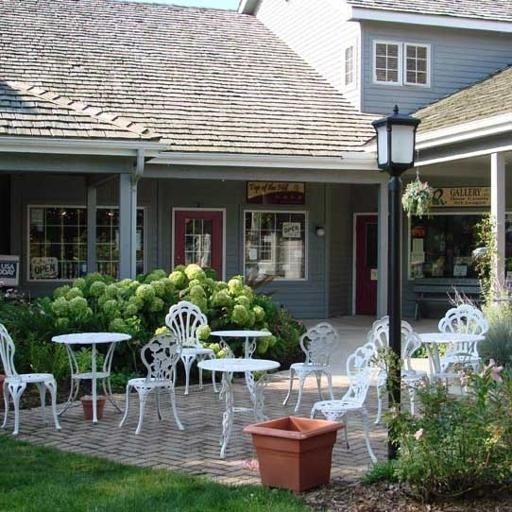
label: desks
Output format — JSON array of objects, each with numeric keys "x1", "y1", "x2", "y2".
[
  {"x1": 413, "y1": 333, "x2": 485, "y2": 389},
  {"x1": 194, "y1": 357, "x2": 281, "y2": 461},
  {"x1": 209, "y1": 329, "x2": 272, "y2": 402},
  {"x1": 52, "y1": 332, "x2": 132, "y2": 416}
]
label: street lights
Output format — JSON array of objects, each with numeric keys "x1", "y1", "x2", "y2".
[{"x1": 371, "y1": 104, "x2": 421, "y2": 461}]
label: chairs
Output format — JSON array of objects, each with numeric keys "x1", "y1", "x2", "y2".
[
  {"x1": 0, "y1": 319, "x2": 61, "y2": 435},
  {"x1": 368, "y1": 315, "x2": 428, "y2": 424},
  {"x1": 165, "y1": 300, "x2": 219, "y2": 397},
  {"x1": 119, "y1": 334, "x2": 184, "y2": 436},
  {"x1": 437, "y1": 305, "x2": 490, "y2": 377},
  {"x1": 310, "y1": 343, "x2": 380, "y2": 465},
  {"x1": 282, "y1": 321, "x2": 334, "y2": 413}
]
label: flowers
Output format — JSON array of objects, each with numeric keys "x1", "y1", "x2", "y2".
[{"x1": 402, "y1": 173, "x2": 434, "y2": 217}]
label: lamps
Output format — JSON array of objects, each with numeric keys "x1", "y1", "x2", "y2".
[{"x1": 316, "y1": 227, "x2": 325, "y2": 237}]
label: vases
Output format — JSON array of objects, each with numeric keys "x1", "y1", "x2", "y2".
[
  {"x1": 79, "y1": 395, "x2": 105, "y2": 422},
  {"x1": 244, "y1": 416, "x2": 346, "y2": 494}
]
